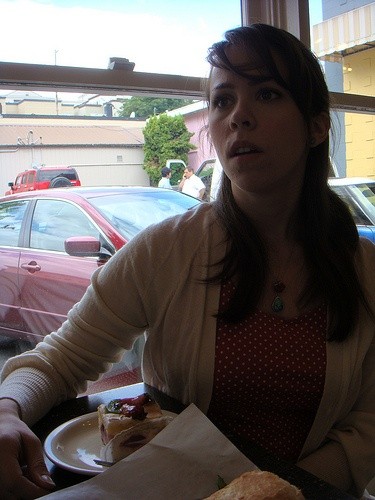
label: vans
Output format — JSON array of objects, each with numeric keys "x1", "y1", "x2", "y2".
[{"x1": 164, "y1": 154, "x2": 339, "y2": 202}]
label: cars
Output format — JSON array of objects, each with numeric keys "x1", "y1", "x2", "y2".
[
  {"x1": 326, "y1": 176, "x2": 375, "y2": 245},
  {"x1": 0, "y1": 186, "x2": 208, "y2": 345}
]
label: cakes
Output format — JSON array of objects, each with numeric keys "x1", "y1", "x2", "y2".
[{"x1": 97, "y1": 392, "x2": 167, "y2": 468}]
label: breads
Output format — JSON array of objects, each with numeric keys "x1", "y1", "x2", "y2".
[{"x1": 206, "y1": 469, "x2": 306, "y2": 500}]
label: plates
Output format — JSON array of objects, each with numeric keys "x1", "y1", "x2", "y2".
[{"x1": 45, "y1": 411, "x2": 179, "y2": 473}]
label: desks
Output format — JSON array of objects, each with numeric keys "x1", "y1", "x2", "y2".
[{"x1": 0, "y1": 381, "x2": 361, "y2": 500}]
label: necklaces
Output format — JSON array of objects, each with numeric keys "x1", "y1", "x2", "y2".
[{"x1": 265, "y1": 243, "x2": 298, "y2": 314}]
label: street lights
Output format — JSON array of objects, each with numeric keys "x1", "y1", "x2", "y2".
[{"x1": 53, "y1": 48, "x2": 60, "y2": 117}]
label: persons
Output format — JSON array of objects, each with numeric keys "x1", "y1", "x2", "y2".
[
  {"x1": 178, "y1": 166, "x2": 207, "y2": 201},
  {"x1": 0, "y1": 23, "x2": 375, "y2": 500},
  {"x1": 158, "y1": 167, "x2": 174, "y2": 190}
]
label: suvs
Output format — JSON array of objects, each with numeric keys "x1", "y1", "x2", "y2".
[{"x1": 4, "y1": 163, "x2": 82, "y2": 197}]
